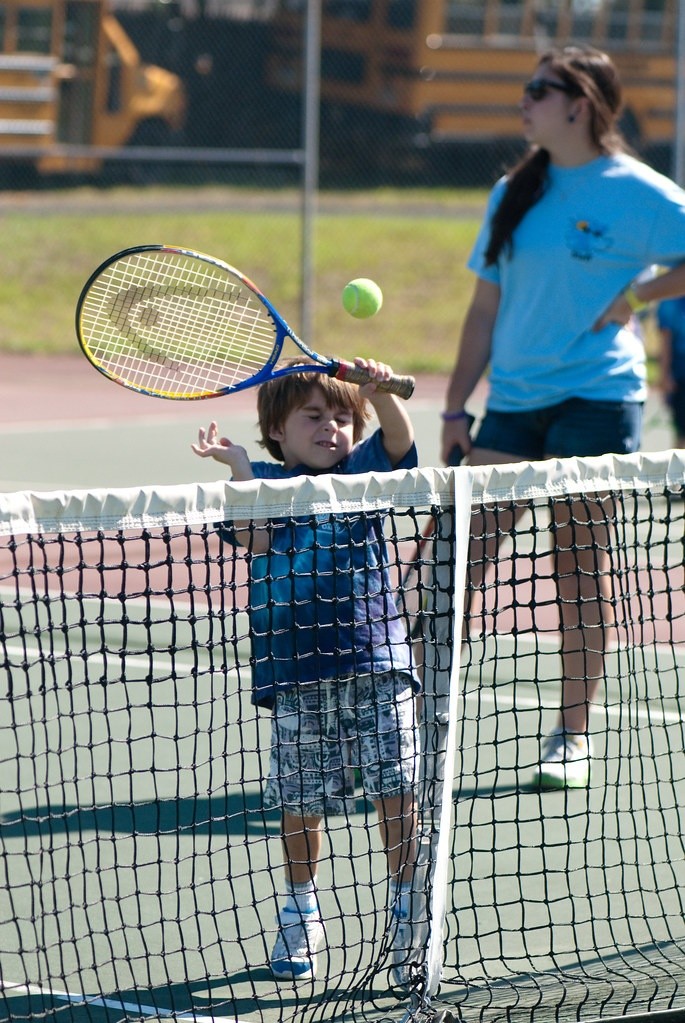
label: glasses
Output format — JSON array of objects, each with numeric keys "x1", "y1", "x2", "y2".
[{"x1": 524, "y1": 78, "x2": 571, "y2": 100}]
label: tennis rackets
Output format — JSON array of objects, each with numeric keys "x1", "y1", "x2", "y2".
[{"x1": 75, "y1": 243, "x2": 416, "y2": 401}]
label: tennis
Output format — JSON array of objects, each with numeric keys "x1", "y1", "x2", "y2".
[{"x1": 342, "y1": 278, "x2": 384, "y2": 321}]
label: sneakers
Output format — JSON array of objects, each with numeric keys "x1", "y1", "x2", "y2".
[
  {"x1": 271, "y1": 906, "x2": 324, "y2": 979},
  {"x1": 389, "y1": 900, "x2": 431, "y2": 992},
  {"x1": 532, "y1": 726, "x2": 594, "y2": 788}
]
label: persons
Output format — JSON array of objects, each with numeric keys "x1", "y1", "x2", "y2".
[
  {"x1": 190, "y1": 355, "x2": 444, "y2": 990},
  {"x1": 410, "y1": 45, "x2": 685, "y2": 789},
  {"x1": 657, "y1": 297, "x2": 685, "y2": 499}
]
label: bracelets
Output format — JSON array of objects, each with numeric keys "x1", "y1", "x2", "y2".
[{"x1": 441, "y1": 409, "x2": 465, "y2": 420}]
